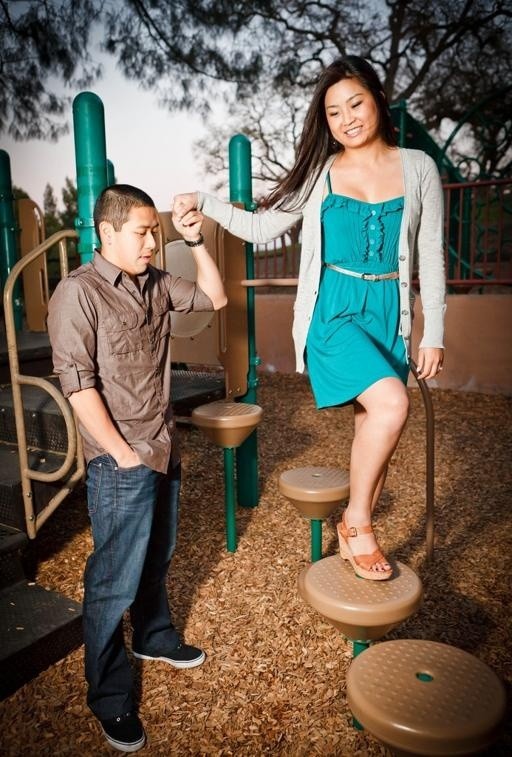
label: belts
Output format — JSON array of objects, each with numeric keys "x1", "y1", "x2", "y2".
[{"x1": 326, "y1": 264, "x2": 399, "y2": 281}]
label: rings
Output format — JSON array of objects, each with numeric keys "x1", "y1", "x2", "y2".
[{"x1": 439, "y1": 366, "x2": 445, "y2": 372}]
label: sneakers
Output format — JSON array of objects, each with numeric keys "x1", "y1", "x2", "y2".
[
  {"x1": 99, "y1": 710, "x2": 147, "y2": 752},
  {"x1": 131, "y1": 640, "x2": 207, "y2": 669}
]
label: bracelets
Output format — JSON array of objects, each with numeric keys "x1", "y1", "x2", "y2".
[{"x1": 182, "y1": 231, "x2": 205, "y2": 247}]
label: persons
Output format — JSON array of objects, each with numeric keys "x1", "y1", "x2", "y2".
[
  {"x1": 168, "y1": 49, "x2": 450, "y2": 585},
  {"x1": 45, "y1": 179, "x2": 234, "y2": 756}
]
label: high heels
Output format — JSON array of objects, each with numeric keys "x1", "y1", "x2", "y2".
[{"x1": 335, "y1": 509, "x2": 393, "y2": 581}]
